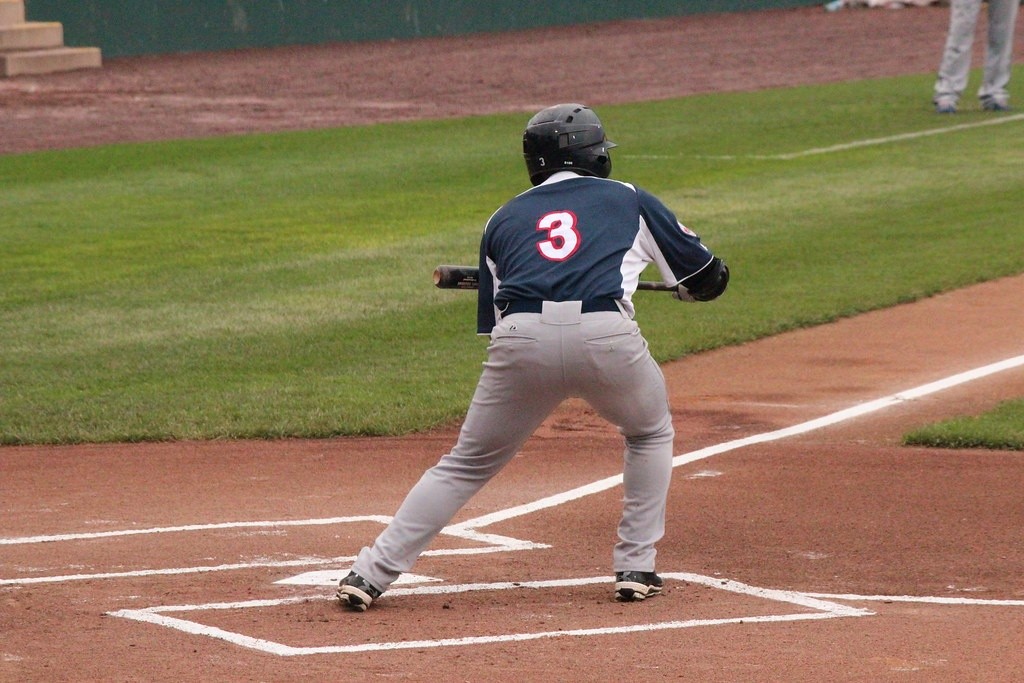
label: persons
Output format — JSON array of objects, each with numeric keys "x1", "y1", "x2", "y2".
[
  {"x1": 335, "y1": 103, "x2": 727, "y2": 611},
  {"x1": 933, "y1": 0, "x2": 1019, "y2": 113}
]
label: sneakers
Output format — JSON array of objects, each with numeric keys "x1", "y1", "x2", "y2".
[
  {"x1": 615, "y1": 570, "x2": 663, "y2": 600},
  {"x1": 336, "y1": 572, "x2": 382, "y2": 612}
]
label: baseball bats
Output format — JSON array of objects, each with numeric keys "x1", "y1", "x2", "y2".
[{"x1": 432, "y1": 265, "x2": 679, "y2": 293}]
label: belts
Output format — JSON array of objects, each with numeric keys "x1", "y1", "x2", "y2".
[{"x1": 501, "y1": 299, "x2": 620, "y2": 314}]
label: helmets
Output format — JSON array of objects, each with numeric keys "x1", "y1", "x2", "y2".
[{"x1": 522, "y1": 104, "x2": 618, "y2": 185}]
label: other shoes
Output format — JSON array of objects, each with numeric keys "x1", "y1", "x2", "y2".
[
  {"x1": 938, "y1": 102, "x2": 957, "y2": 113},
  {"x1": 983, "y1": 99, "x2": 1011, "y2": 110}
]
